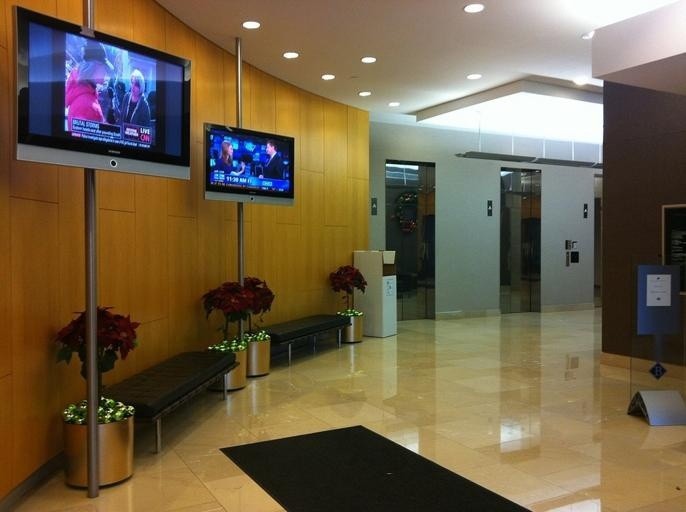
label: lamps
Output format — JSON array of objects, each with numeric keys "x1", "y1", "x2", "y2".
[{"x1": 415, "y1": 79, "x2": 604, "y2": 147}]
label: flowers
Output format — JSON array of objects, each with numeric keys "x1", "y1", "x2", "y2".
[
  {"x1": 47, "y1": 302, "x2": 137, "y2": 421},
  {"x1": 242, "y1": 273, "x2": 270, "y2": 340},
  {"x1": 207, "y1": 278, "x2": 247, "y2": 345},
  {"x1": 330, "y1": 266, "x2": 362, "y2": 316}
]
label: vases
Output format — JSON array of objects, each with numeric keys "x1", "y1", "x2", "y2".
[
  {"x1": 207, "y1": 343, "x2": 246, "y2": 394},
  {"x1": 338, "y1": 310, "x2": 365, "y2": 344},
  {"x1": 62, "y1": 415, "x2": 133, "y2": 491},
  {"x1": 245, "y1": 339, "x2": 270, "y2": 380}
]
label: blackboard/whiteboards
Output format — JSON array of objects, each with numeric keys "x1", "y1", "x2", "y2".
[{"x1": 661, "y1": 204, "x2": 686, "y2": 295}]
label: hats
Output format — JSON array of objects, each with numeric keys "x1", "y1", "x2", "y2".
[{"x1": 83, "y1": 39, "x2": 115, "y2": 71}]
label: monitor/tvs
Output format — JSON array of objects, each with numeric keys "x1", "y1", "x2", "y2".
[
  {"x1": 12, "y1": 5, "x2": 191, "y2": 182},
  {"x1": 202, "y1": 122, "x2": 294, "y2": 206}
]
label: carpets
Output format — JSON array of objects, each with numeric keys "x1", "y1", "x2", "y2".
[{"x1": 221, "y1": 425, "x2": 532, "y2": 511}]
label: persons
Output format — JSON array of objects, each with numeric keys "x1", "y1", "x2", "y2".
[
  {"x1": 64, "y1": 37, "x2": 116, "y2": 132},
  {"x1": 117, "y1": 68, "x2": 149, "y2": 126},
  {"x1": 212, "y1": 139, "x2": 245, "y2": 176},
  {"x1": 258, "y1": 139, "x2": 286, "y2": 178}
]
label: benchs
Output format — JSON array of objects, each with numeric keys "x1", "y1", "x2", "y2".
[
  {"x1": 246, "y1": 313, "x2": 350, "y2": 363},
  {"x1": 102, "y1": 351, "x2": 237, "y2": 450}
]
